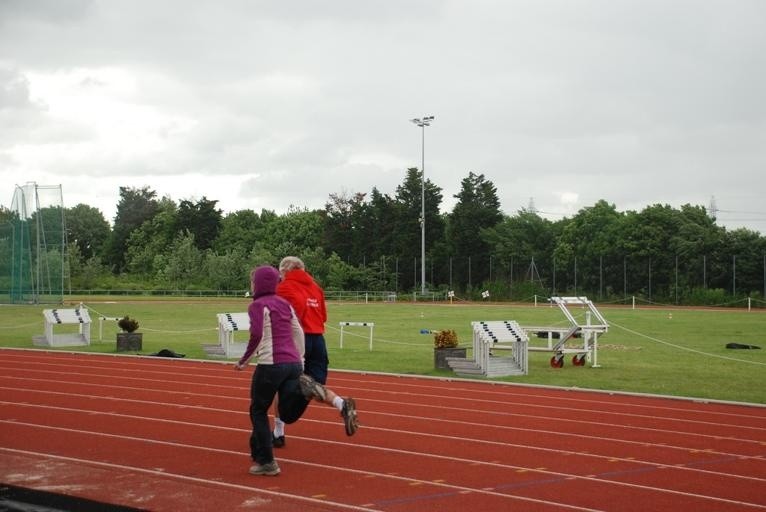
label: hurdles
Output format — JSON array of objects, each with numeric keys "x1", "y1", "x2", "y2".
[
  {"x1": 216, "y1": 313, "x2": 258, "y2": 359},
  {"x1": 43, "y1": 308, "x2": 92, "y2": 348},
  {"x1": 340, "y1": 322, "x2": 375, "y2": 352},
  {"x1": 471, "y1": 320, "x2": 528, "y2": 377},
  {"x1": 99, "y1": 317, "x2": 124, "y2": 343}
]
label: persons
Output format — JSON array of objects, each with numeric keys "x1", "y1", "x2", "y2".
[
  {"x1": 233, "y1": 265, "x2": 326, "y2": 476},
  {"x1": 270, "y1": 255, "x2": 359, "y2": 447}
]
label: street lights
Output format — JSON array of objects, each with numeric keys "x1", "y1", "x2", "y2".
[{"x1": 408, "y1": 112, "x2": 436, "y2": 299}]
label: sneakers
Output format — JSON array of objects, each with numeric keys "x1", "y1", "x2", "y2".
[
  {"x1": 270, "y1": 432, "x2": 286, "y2": 448},
  {"x1": 248, "y1": 461, "x2": 280, "y2": 478},
  {"x1": 340, "y1": 398, "x2": 359, "y2": 435},
  {"x1": 299, "y1": 373, "x2": 327, "y2": 403}
]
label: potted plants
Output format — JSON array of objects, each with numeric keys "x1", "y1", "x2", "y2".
[
  {"x1": 433, "y1": 329, "x2": 466, "y2": 370},
  {"x1": 114, "y1": 315, "x2": 142, "y2": 352}
]
